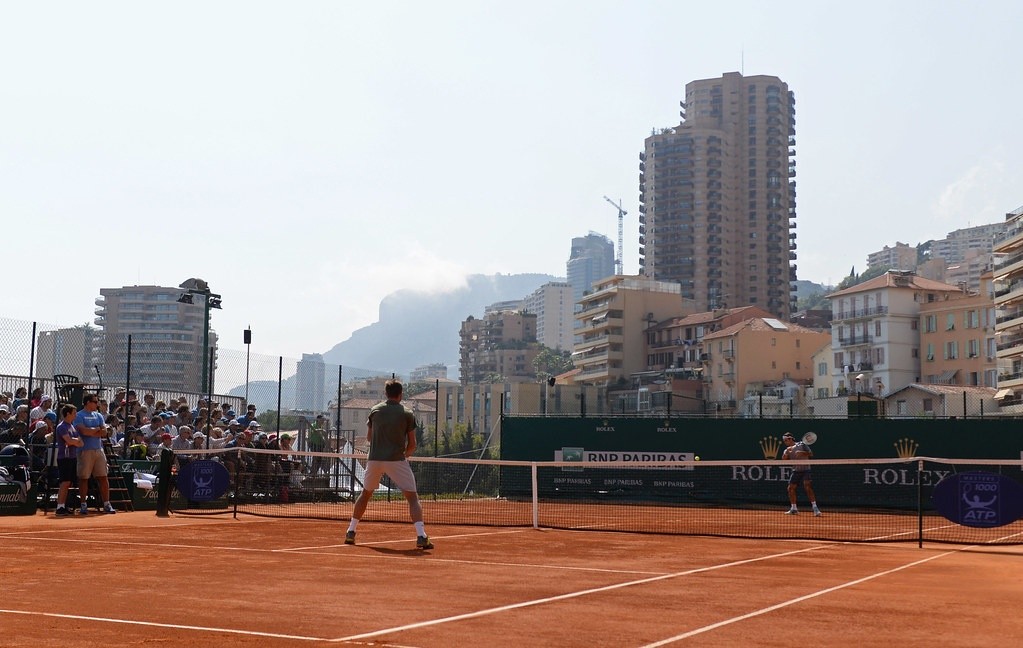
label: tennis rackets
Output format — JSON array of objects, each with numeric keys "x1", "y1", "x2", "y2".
[{"x1": 789, "y1": 432, "x2": 818, "y2": 452}]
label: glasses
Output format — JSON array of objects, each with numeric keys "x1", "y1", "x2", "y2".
[
  {"x1": 89, "y1": 400, "x2": 98, "y2": 405},
  {"x1": 253, "y1": 426, "x2": 259, "y2": 429},
  {"x1": 142, "y1": 410, "x2": 148, "y2": 414}
]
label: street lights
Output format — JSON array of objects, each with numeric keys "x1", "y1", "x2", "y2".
[
  {"x1": 876, "y1": 379, "x2": 882, "y2": 414},
  {"x1": 546, "y1": 374, "x2": 557, "y2": 415},
  {"x1": 177, "y1": 289, "x2": 223, "y2": 393}
]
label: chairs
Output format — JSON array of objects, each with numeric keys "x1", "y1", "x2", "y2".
[{"x1": 44, "y1": 375, "x2": 134, "y2": 513}]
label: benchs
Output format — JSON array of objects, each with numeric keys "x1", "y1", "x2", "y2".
[{"x1": 0, "y1": 405, "x2": 350, "y2": 501}]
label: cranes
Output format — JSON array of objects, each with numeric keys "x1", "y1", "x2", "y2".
[{"x1": 604, "y1": 195, "x2": 628, "y2": 275}]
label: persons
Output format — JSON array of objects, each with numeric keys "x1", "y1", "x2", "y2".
[
  {"x1": 74, "y1": 394, "x2": 118, "y2": 514},
  {"x1": 0, "y1": 386, "x2": 308, "y2": 490},
  {"x1": 156, "y1": 433, "x2": 176, "y2": 518},
  {"x1": 307, "y1": 414, "x2": 327, "y2": 477},
  {"x1": 782, "y1": 432, "x2": 821, "y2": 517},
  {"x1": 57, "y1": 404, "x2": 84, "y2": 515},
  {"x1": 344, "y1": 378, "x2": 435, "y2": 549}
]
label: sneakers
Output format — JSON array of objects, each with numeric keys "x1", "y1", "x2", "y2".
[
  {"x1": 813, "y1": 506, "x2": 822, "y2": 517},
  {"x1": 417, "y1": 535, "x2": 434, "y2": 549},
  {"x1": 344, "y1": 530, "x2": 356, "y2": 544},
  {"x1": 784, "y1": 509, "x2": 799, "y2": 515},
  {"x1": 104, "y1": 502, "x2": 116, "y2": 514},
  {"x1": 55, "y1": 507, "x2": 68, "y2": 515},
  {"x1": 80, "y1": 504, "x2": 87, "y2": 514}
]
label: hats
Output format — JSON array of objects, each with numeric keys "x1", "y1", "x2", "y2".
[
  {"x1": 119, "y1": 399, "x2": 126, "y2": 405},
  {"x1": 204, "y1": 398, "x2": 219, "y2": 404},
  {"x1": 237, "y1": 430, "x2": 252, "y2": 439},
  {"x1": 316, "y1": 415, "x2": 323, "y2": 418},
  {"x1": 179, "y1": 426, "x2": 207, "y2": 439},
  {"x1": 113, "y1": 386, "x2": 127, "y2": 395},
  {"x1": 222, "y1": 404, "x2": 240, "y2": 425},
  {"x1": 128, "y1": 425, "x2": 148, "y2": 436},
  {"x1": 161, "y1": 433, "x2": 174, "y2": 441},
  {"x1": 33, "y1": 395, "x2": 56, "y2": 433},
  {"x1": 0, "y1": 404, "x2": 9, "y2": 412},
  {"x1": 154, "y1": 396, "x2": 188, "y2": 419},
  {"x1": 259, "y1": 432, "x2": 292, "y2": 445},
  {"x1": 248, "y1": 404, "x2": 265, "y2": 427},
  {"x1": 16, "y1": 405, "x2": 28, "y2": 412}
]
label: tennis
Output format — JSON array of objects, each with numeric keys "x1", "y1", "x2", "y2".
[{"x1": 695, "y1": 456, "x2": 699, "y2": 460}]
